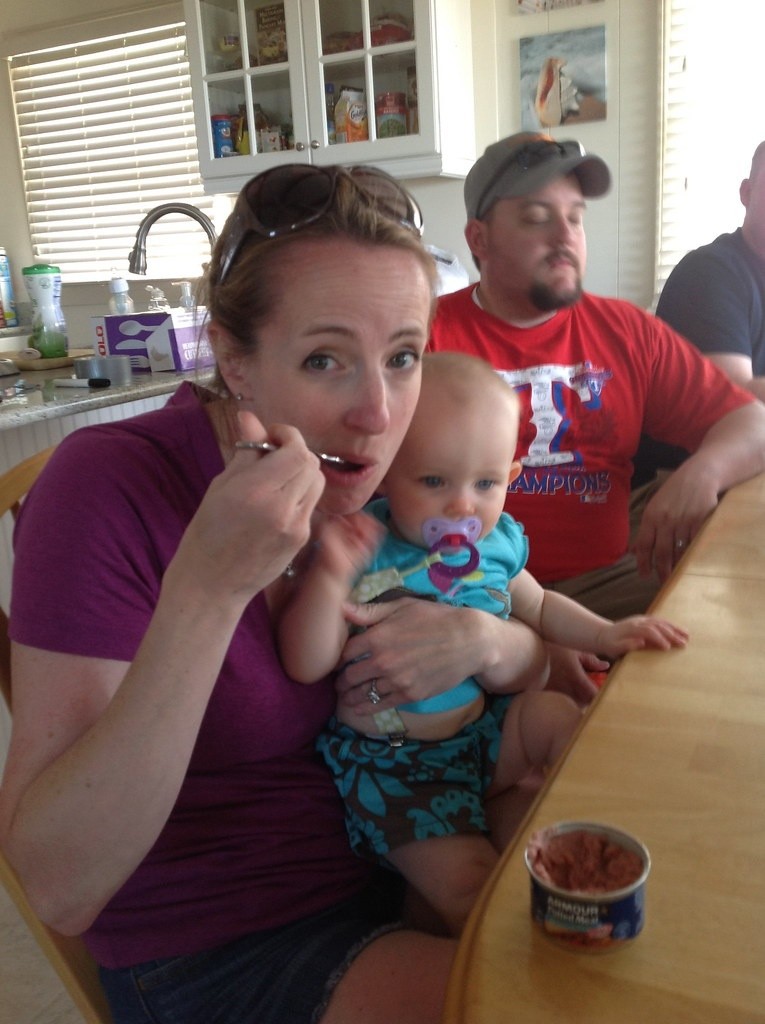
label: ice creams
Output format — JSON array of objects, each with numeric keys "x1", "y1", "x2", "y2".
[{"x1": 524, "y1": 822, "x2": 652, "y2": 955}]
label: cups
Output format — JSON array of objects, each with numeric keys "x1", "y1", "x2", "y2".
[
  {"x1": 210, "y1": 114, "x2": 233, "y2": 158},
  {"x1": 218, "y1": 31, "x2": 240, "y2": 52},
  {"x1": 21, "y1": 264, "x2": 69, "y2": 358},
  {"x1": 235, "y1": 129, "x2": 263, "y2": 155}
]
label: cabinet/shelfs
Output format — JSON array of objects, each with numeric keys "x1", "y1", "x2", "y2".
[{"x1": 183, "y1": 0, "x2": 477, "y2": 201}]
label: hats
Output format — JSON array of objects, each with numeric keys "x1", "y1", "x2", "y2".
[{"x1": 464, "y1": 132, "x2": 610, "y2": 221}]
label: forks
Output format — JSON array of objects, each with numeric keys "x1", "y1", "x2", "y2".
[{"x1": 129, "y1": 355, "x2": 173, "y2": 369}]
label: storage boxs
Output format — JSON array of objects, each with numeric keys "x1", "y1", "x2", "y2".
[{"x1": 90, "y1": 306, "x2": 216, "y2": 374}]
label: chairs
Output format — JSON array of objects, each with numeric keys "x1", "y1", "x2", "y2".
[{"x1": 0, "y1": 447, "x2": 111, "y2": 1024}]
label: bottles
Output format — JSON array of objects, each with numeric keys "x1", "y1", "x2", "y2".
[
  {"x1": 238, "y1": 103, "x2": 266, "y2": 130},
  {"x1": 326, "y1": 82, "x2": 336, "y2": 144},
  {"x1": 145, "y1": 283, "x2": 171, "y2": 312},
  {"x1": 106, "y1": 276, "x2": 135, "y2": 317}
]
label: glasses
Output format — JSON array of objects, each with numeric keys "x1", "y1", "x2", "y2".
[
  {"x1": 217, "y1": 164, "x2": 425, "y2": 288},
  {"x1": 475, "y1": 140, "x2": 586, "y2": 219}
]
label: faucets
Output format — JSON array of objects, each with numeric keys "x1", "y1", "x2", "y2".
[{"x1": 126, "y1": 202, "x2": 218, "y2": 275}]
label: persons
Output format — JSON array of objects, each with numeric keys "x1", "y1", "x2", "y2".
[
  {"x1": 282, "y1": 352, "x2": 690, "y2": 923},
  {"x1": 626, "y1": 140, "x2": 765, "y2": 549},
  {"x1": 1, "y1": 163, "x2": 552, "y2": 1024},
  {"x1": 423, "y1": 131, "x2": 765, "y2": 709}
]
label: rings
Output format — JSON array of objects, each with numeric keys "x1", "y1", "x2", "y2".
[
  {"x1": 368, "y1": 680, "x2": 383, "y2": 704},
  {"x1": 675, "y1": 541, "x2": 687, "y2": 547}
]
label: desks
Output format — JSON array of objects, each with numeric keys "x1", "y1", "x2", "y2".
[
  {"x1": 0, "y1": 366, "x2": 216, "y2": 618},
  {"x1": 444, "y1": 473, "x2": 765, "y2": 1024}
]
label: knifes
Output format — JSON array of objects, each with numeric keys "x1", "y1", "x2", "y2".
[{"x1": 115, "y1": 340, "x2": 171, "y2": 351}]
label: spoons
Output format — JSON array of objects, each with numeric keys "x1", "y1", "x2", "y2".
[{"x1": 118, "y1": 320, "x2": 170, "y2": 336}]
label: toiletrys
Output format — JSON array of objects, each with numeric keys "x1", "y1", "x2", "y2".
[
  {"x1": 170, "y1": 281, "x2": 195, "y2": 307},
  {"x1": 144, "y1": 284, "x2": 171, "y2": 311},
  {"x1": 107, "y1": 264, "x2": 133, "y2": 314}
]
